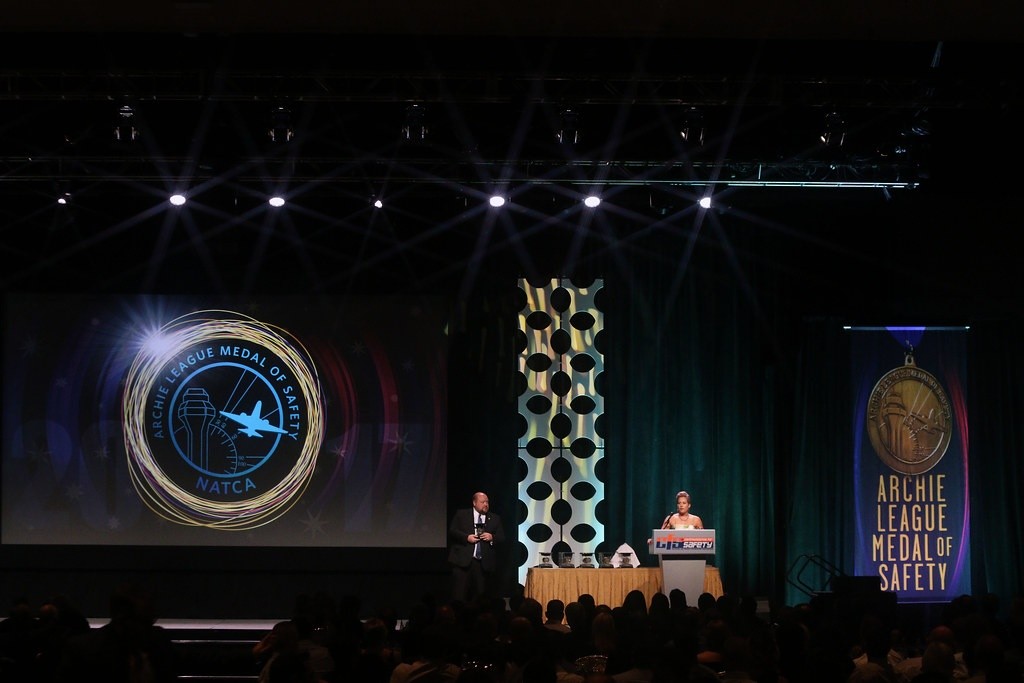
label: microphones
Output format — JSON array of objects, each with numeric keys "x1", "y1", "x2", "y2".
[{"x1": 665, "y1": 512, "x2": 673, "y2": 524}]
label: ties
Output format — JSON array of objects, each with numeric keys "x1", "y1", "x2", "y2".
[{"x1": 476, "y1": 513, "x2": 483, "y2": 560}]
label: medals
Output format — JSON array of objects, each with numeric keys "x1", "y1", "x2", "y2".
[{"x1": 868, "y1": 366, "x2": 953, "y2": 475}]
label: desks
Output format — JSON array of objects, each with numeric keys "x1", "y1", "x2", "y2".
[{"x1": 524, "y1": 566, "x2": 723, "y2": 626}]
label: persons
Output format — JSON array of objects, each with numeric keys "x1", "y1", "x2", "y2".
[
  {"x1": 450, "y1": 492, "x2": 505, "y2": 608},
  {"x1": 0, "y1": 588, "x2": 1024, "y2": 683},
  {"x1": 648, "y1": 491, "x2": 703, "y2": 544}
]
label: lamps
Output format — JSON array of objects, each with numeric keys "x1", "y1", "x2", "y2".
[
  {"x1": 57, "y1": 183, "x2": 718, "y2": 210},
  {"x1": 55, "y1": 98, "x2": 938, "y2": 149}
]
